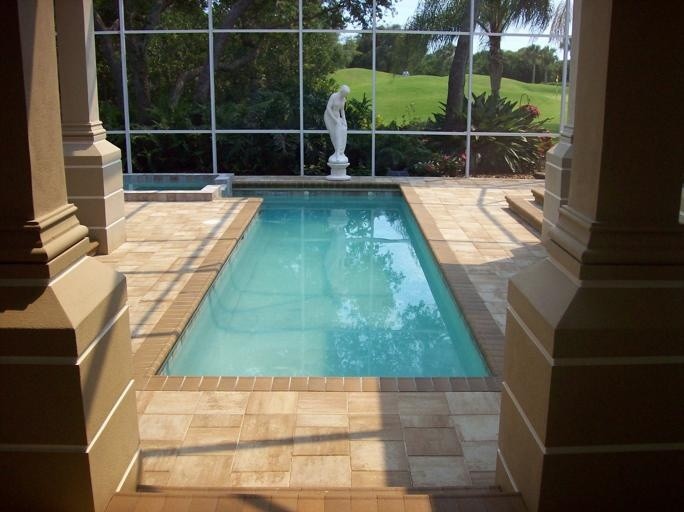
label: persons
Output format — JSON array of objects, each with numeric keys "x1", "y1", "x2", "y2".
[{"x1": 324, "y1": 84, "x2": 350, "y2": 165}]
